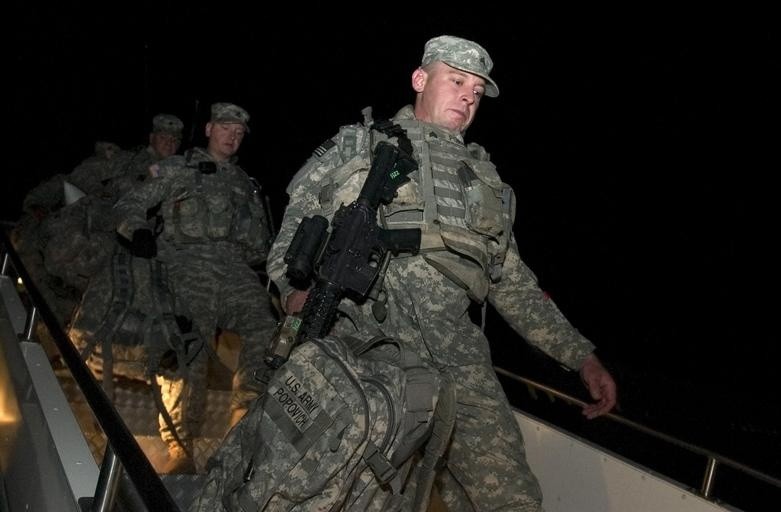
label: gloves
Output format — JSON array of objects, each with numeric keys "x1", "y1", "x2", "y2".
[{"x1": 130, "y1": 229, "x2": 157, "y2": 257}]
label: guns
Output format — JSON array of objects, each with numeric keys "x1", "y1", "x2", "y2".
[{"x1": 282, "y1": 142, "x2": 420, "y2": 363}]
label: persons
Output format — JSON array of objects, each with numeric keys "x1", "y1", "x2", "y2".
[
  {"x1": 259, "y1": 31, "x2": 618, "y2": 510},
  {"x1": 111, "y1": 97, "x2": 277, "y2": 481},
  {"x1": 8, "y1": 202, "x2": 52, "y2": 286},
  {"x1": 68, "y1": 111, "x2": 184, "y2": 388},
  {"x1": 22, "y1": 142, "x2": 123, "y2": 369}
]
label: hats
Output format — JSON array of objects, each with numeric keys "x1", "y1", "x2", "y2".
[
  {"x1": 210, "y1": 102, "x2": 249, "y2": 132},
  {"x1": 422, "y1": 33, "x2": 492, "y2": 100},
  {"x1": 151, "y1": 114, "x2": 181, "y2": 138}
]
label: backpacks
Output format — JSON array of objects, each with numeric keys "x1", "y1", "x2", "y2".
[
  {"x1": 69, "y1": 255, "x2": 207, "y2": 381},
  {"x1": 191, "y1": 337, "x2": 456, "y2": 512}
]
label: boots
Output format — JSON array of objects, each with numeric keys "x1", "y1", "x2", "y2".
[{"x1": 164, "y1": 443, "x2": 197, "y2": 474}]
